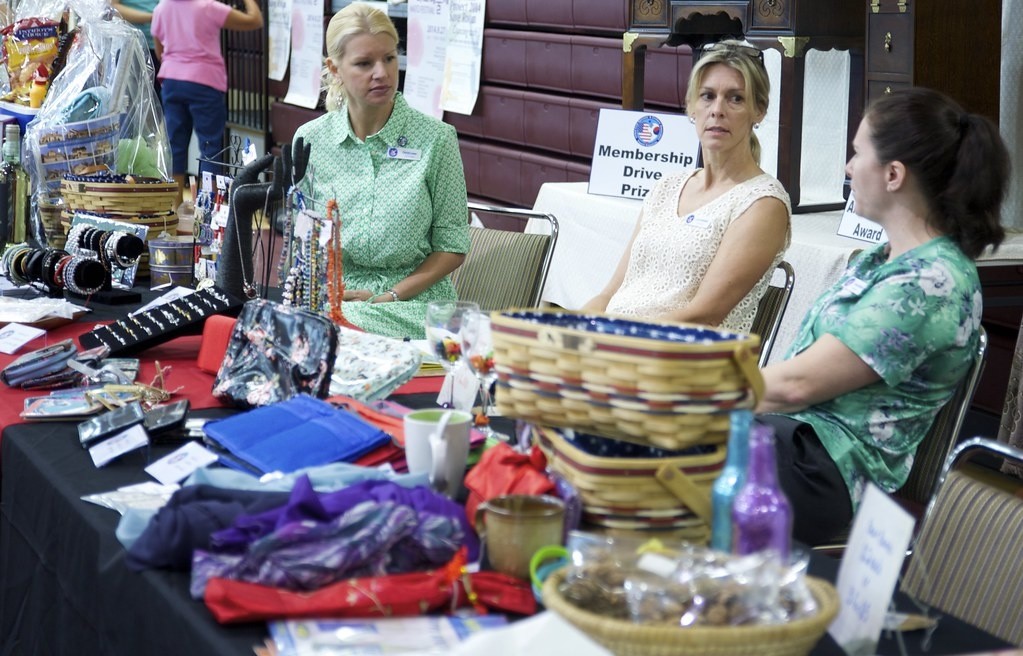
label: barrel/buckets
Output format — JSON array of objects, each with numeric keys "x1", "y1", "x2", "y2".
[{"x1": 149, "y1": 241, "x2": 194, "y2": 289}]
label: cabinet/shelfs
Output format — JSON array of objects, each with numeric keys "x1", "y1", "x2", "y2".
[{"x1": 865, "y1": 0, "x2": 1001, "y2": 115}]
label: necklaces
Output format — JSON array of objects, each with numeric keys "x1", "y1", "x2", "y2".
[
  {"x1": 275, "y1": 184, "x2": 346, "y2": 323},
  {"x1": 228, "y1": 180, "x2": 275, "y2": 299}
]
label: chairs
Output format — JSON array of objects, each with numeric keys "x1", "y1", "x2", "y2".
[
  {"x1": 449, "y1": 203, "x2": 556, "y2": 310},
  {"x1": 751, "y1": 260, "x2": 1023, "y2": 655}
]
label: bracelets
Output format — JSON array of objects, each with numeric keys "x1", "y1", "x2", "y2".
[
  {"x1": 64, "y1": 222, "x2": 137, "y2": 269},
  {"x1": 385, "y1": 289, "x2": 400, "y2": 302},
  {"x1": 0, "y1": 244, "x2": 105, "y2": 296}
]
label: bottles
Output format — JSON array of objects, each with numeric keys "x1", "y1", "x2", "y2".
[
  {"x1": 30, "y1": 80, "x2": 48, "y2": 108},
  {"x1": 731, "y1": 426, "x2": 792, "y2": 563},
  {"x1": 5, "y1": 124, "x2": 20, "y2": 164},
  {"x1": 711, "y1": 411, "x2": 754, "y2": 554}
]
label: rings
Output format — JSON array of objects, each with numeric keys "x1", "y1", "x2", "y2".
[
  {"x1": 104, "y1": 324, "x2": 127, "y2": 345},
  {"x1": 127, "y1": 312, "x2": 153, "y2": 335},
  {"x1": 115, "y1": 318, "x2": 138, "y2": 340},
  {"x1": 141, "y1": 310, "x2": 165, "y2": 331},
  {"x1": 153, "y1": 305, "x2": 178, "y2": 326},
  {"x1": 166, "y1": 296, "x2": 205, "y2": 321},
  {"x1": 191, "y1": 291, "x2": 218, "y2": 310},
  {"x1": 201, "y1": 286, "x2": 229, "y2": 306},
  {"x1": 90, "y1": 331, "x2": 112, "y2": 351}
]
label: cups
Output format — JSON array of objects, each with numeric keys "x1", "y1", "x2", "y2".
[
  {"x1": 404, "y1": 409, "x2": 473, "y2": 500},
  {"x1": 474, "y1": 494, "x2": 566, "y2": 582}
]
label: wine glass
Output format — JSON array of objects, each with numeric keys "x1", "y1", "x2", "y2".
[
  {"x1": 459, "y1": 312, "x2": 498, "y2": 440},
  {"x1": 425, "y1": 301, "x2": 480, "y2": 409}
]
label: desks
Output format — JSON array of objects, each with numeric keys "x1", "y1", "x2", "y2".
[{"x1": 523, "y1": 181, "x2": 1022, "y2": 366}]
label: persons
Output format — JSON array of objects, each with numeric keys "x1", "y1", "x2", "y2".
[
  {"x1": 108, "y1": 0, "x2": 166, "y2": 140},
  {"x1": 151, "y1": 0, "x2": 266, "y2": 216},
  {"x1": 290, "y1": 3, "x2": 470, "y2": 338},
  {"x1": 752, "y1": 88, "x2": 1016, "y2": 549},
  {"x1": 214, "y1": 134, "x2": 312, "y2": 309},
  {"x1": 581, "y1": 50, "x2": 793, "y2": 335}
]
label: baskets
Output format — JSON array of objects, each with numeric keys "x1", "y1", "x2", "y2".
[
  {"x1": 564, "y1": 507, "x2": 716, "y2": 566},
  {"x1": 31, "y1": 111, "x2": 180, "y2": 278},
  {"x1": 536, "y1": 544, "x2": 844, "y2": 655},
  {"x1": 528, "y1": 423, "x2": 727, "y2": 523},
  {"x1": 483, "y1": 304, "x2": 768, "y2": 448}
]
